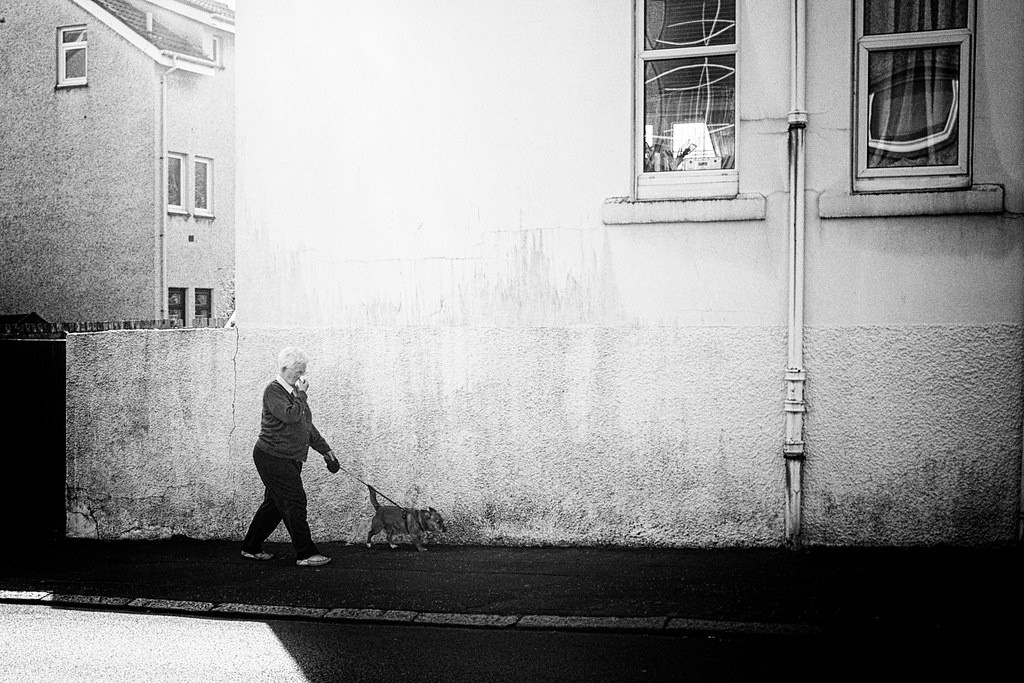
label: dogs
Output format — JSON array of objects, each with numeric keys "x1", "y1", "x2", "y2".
[{"x1": 366, "y1": 484, "x2": 449, "y2": 553}]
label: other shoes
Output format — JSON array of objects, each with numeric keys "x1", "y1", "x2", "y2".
[
  {"x1": 241, "y1": 550, "x2": 273, "y2": 560},
  {"x1": 296, "y1": 554, "x2": 332, "y2": 566}
]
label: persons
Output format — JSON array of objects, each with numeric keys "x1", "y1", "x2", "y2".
[{"x1": 240, "y1": 348, "x2": 343, "y2": 567}]
